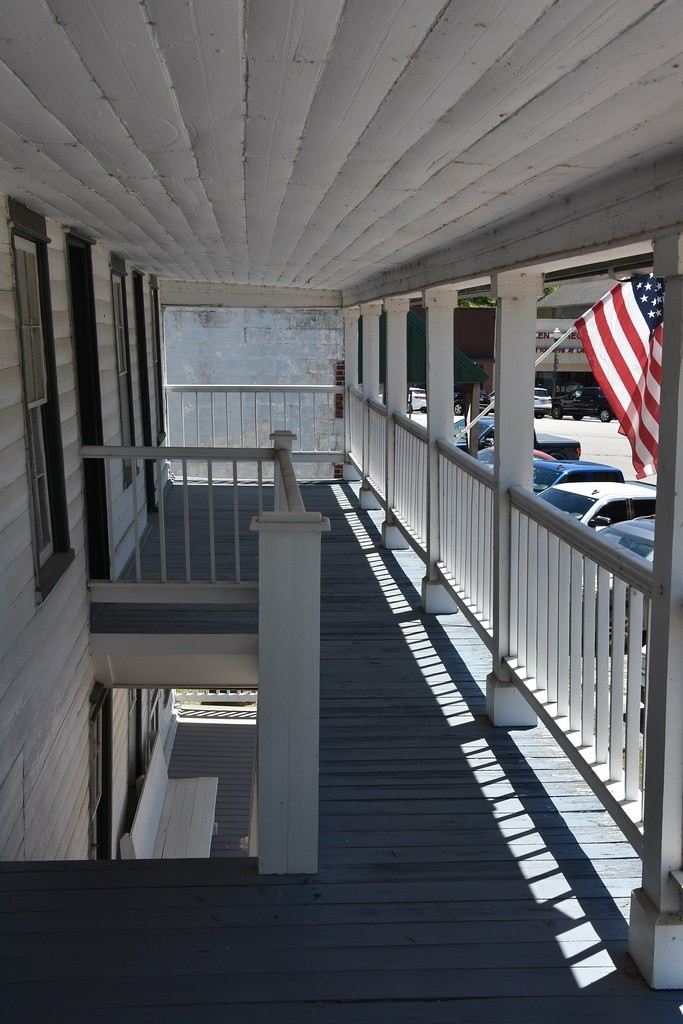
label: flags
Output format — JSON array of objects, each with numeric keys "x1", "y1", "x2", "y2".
[{"x1": 575, "y1": 275, "x2": 665, "y2": 479}]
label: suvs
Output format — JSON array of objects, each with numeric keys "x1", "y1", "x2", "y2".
[
  {"x1": 488, "y1": 387, "x2": 553, "y2": 419},
  {"x1": 454, "y1": 383, "x2": 495, "y2": 416}
]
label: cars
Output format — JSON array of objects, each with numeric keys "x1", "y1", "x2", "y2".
[
  {"x1": 379, "y1": 387, "x2": 427, "y2": 414},
  {"x1": 574, "y1": 515, "x2": 656, "y2": 657},
  {"x1": 469, "y1": 445, "x2": 657, "y2": 533}
]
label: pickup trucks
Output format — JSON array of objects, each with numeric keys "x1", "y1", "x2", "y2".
[
  {"x1": 449, "y1": 411, "x2": 582, "y2": 460},
  {"x1": 551, "y1": 386, "x2": 618, "y2": 423}
]
